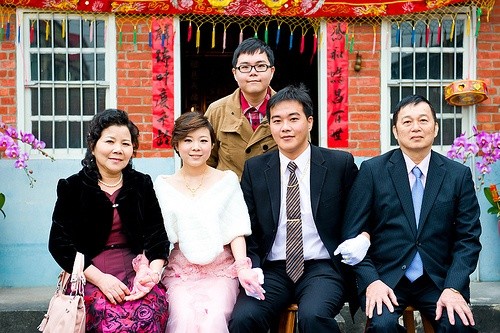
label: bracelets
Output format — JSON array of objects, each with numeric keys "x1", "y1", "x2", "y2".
[
  {"x1": 449, "y1": 288, "x2": 459, "y2": 293},
  {"x1": 153, "y1": 271, "x2": 161, "y2": 284}
]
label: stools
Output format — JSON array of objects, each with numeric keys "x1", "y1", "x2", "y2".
[
  {"x1": 403, "y1": 307, "x2": 431, "y2": 333},
  {"x1": 286, "y1": 305, "x2": 298, "y2": 333}
]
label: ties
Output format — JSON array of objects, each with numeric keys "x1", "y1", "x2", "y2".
[
  {"x1": 405, "y1": 166, "x2": 425, "y2": 283},
  {"x1": 285, "y1": 161, "x2": 305, "y2": 283}
]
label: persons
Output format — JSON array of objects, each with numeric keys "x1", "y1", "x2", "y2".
[
  {"x1": 336, "y1": 95, "x2": 483, "y2": 333},
  {"x1": 48, "y1": 108, "x2": 170, "y2": 333},
  {"x1": 154, "y1": 112, "x2": 265, "y2": 333},
  {"x1": 229, "y1": 84, "x2": 371, "y2": 333},
  {"x1": 204, "y1": 38, "x2": 312, "y2": 182}
]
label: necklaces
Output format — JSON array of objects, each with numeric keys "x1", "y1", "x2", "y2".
[
  {"x1": 98, "y1": 173, "x2": 123, "y2": 187},
  {"x1": 237, "y1": 266, "x2": 249, "y2": 273},
  {"x1": 280, "y1": 160, "x2": 310, "y2": 187},
  {"x1": 185, "y1": 176, "x2": 203, "y2": 196}
]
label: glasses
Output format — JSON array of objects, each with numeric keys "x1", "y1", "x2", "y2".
[{"x1": 235, "y1": 63, "x2": 274, "y2": 73}]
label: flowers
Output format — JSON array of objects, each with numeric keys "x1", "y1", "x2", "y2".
[
  {"x1": 0, "y1": 122, "x2": 55, "y2": 188},
  {"x1": 447, "y1": 125, "x2": 500, "y2": 191}
]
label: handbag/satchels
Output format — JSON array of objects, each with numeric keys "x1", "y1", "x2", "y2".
[{"x1": 36, "y1": 252, "x2": 86, "y2": 333}]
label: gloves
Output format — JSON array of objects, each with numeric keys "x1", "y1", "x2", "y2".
[
  {"x1": 245, "y1": 267, "x2": 266, "y2": 300},
  {"x1": 333, "y1": 234, "x2": 371, "y2": 266}
]
